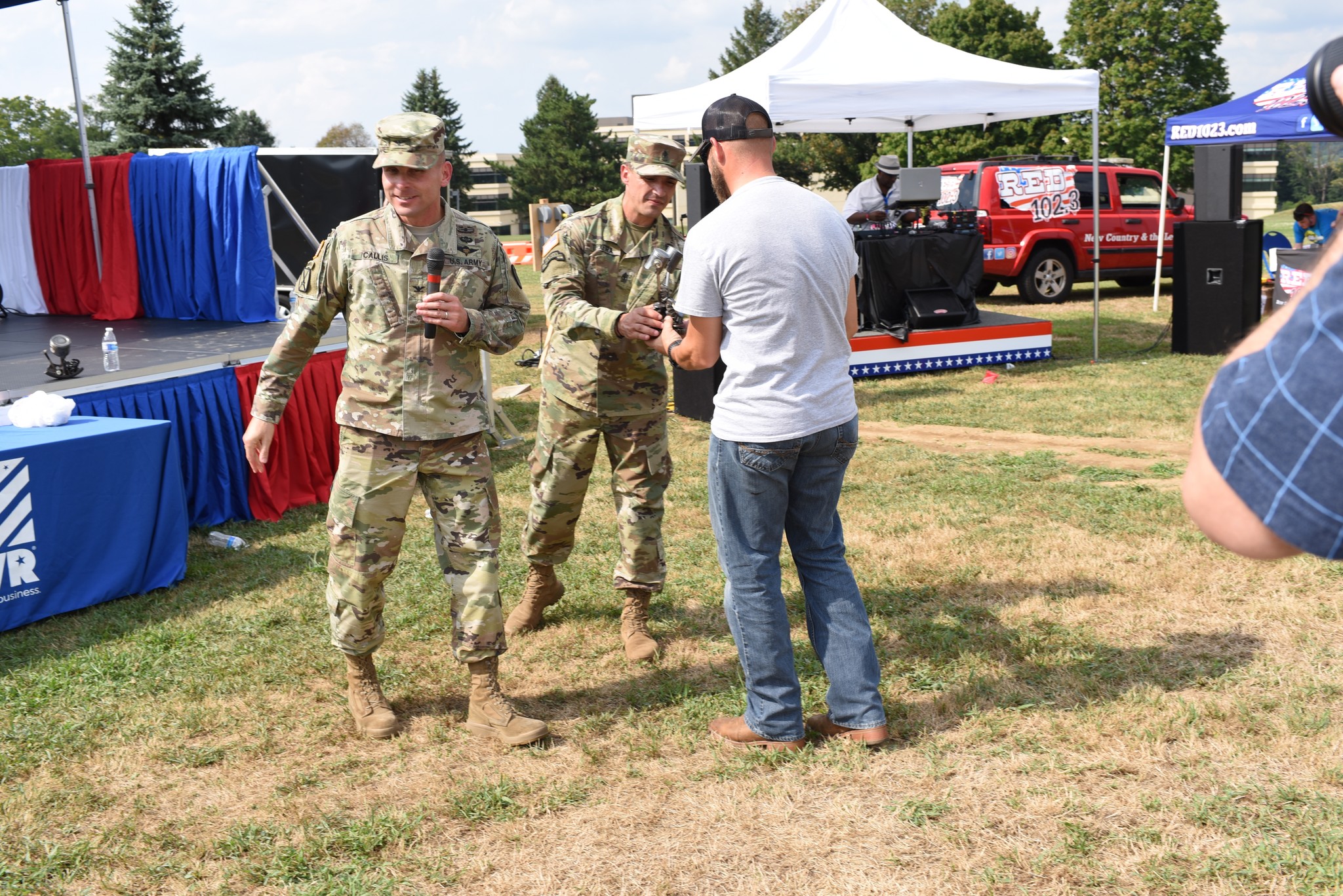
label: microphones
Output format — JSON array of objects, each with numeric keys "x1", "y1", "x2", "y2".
[{"x1": 424, "y1": 247, "x2": 444, "y2": 339}]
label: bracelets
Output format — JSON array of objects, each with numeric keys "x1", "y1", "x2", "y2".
[
  {"x1": 617, "y1": 315, "x2": 625, "y2": 337},
  {"x1": 668, "y1": 339, "x2": 686, "y2": 370},
  {"x1": 866, "y1": 212, "x2": 869, "y2": 220}
]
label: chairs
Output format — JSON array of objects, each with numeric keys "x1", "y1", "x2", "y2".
[{"x1": 1262, "y1": 231, "x2": 1293, "y2": 281}]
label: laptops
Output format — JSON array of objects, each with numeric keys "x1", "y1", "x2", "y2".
[{"x1": 882, "y1": 167, "x2": 941, "y2": 209}]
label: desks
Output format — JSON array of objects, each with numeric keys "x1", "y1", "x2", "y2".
[
  {"x1": 854, "y1": 229, "x2": 984, "y2": 343},
  {"x1": 1272, "y1": 248, "x2": 1327, "y2": 315},
  {"x1": 0, "y1": 416, "x2": 187, "y2": 638}
]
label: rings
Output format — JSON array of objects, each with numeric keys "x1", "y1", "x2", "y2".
[{"x1": 445, "y1": 311, "x2": 448, "y2": 319}]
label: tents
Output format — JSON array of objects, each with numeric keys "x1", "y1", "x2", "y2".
[
  {"x1": 631, "y1": 0, "x2": 1099, "y2": 361},
  {"x1": 1152, "y1": 59, "x2": 1342, "y2": 312}
]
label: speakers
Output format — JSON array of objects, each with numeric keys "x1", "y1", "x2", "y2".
[
  {"x1": 686, "y1": 162, "x2": 720, "y2": 232},
  {"x1": 1171, "y1": 143, "x2": 1264, "y2": 356},
  {"x1": 673, "y1": 356, "x2": 727, "y2": 424},
  {"x1": 904, "y1": 287, "x2": 967, "y2": 329}
]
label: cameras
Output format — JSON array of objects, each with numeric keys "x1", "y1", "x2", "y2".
[{"x1": 1306, "y1": 36, "x2": 1343, "y2": 136}]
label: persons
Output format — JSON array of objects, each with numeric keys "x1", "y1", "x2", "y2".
[
  {"x1": 1292, "y1": 203, "x2": 1340, "y2": 250},
  {"x1": 505, "y1": 135, "x2": 686, "y2": 664},
  {"x1": 642, "y1": 94, "x2": 891, "y2": 749},
  {"x1": 1182, "y1": 219, "x2": 1343, "y2": 562},
  {"x1": 242, "y1": 112, "x2": 548, "y2": 745},
  {"x1": 842, "y1": 154, "x2": 931, "y2": 233}
]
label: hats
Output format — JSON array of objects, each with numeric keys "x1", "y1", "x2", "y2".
[
  {"x1": 372, "y1": 112, "x2": 444, "y2": 169},
  {"x1": 625, "y1": 134, "x2": 686, "y2": 186},
  {"x1": 688, "y1": 93, "x2": 772, "y2": 164},
  {"x1": 875, "y1": 155, "x2": 901, "y2": 175}
]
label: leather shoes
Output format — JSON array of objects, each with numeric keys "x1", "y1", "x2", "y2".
[
  {"x1": 805, "y1": 714, "x2": 888, "y2": 744},
  {"x1": 710, "y1": 714, "x2": 805, "y2": 752}
]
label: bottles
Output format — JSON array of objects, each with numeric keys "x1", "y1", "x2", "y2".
[
  {"x1": 101, "y1": 327, "x2": 120, "y2": 372},
  {"x1": 289, "y1": 287, "x2": 296, "y2": 313}
]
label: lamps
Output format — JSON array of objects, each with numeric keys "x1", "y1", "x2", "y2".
[{"x1": 42, "y1": 335, "x2": 84, "y2": 379}]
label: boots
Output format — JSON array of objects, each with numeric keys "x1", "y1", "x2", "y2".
[
  {"x1": 467, "y1": 656, "x2": 548, "y2": 747},
  {"x1": 503, "y1": 563, "x2": 565, "y2": 636},
  {"x1": 620, "y1": 589, "x2": 659, "y2": 659},
  {"x1": 344, "y1": 652, "x2": 397, "y2": 737}
]
